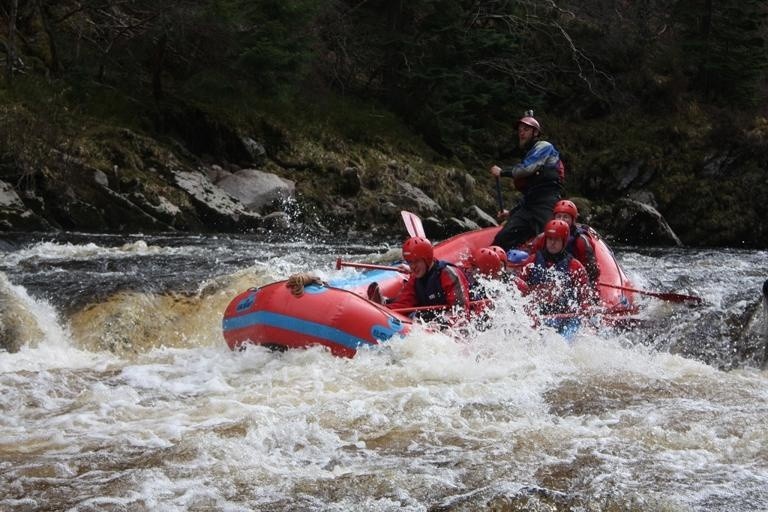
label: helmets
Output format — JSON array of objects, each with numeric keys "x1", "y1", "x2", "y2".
[
  {"x1": 544, "y1": 219, "x2": 570, "y2": 247},
  {"x1": 402, "y1": 237, "x2": 434, "y2": 267},
  {"x1": 520, "y1": 116, "x2": 540, "y2": 130},
  {"x1": 488, "y1": 245, "x2": 508, "y2": 263},
  {"x1": 507, "y1": 248, "x2": 529, "y2": 264},
  {"x1": 474, "y1": 248, "x2": 501, "y2": 280},
  {"x1": 552, "y1": 199, "x2": 577, "y2": 218}
]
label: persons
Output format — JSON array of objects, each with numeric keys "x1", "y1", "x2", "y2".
[
  {"x1": 366, "y1": 200, "x2": 601, "y2": 347},
  {"x1": 489, "y1": 117, "x2": 564, "y2": 256}
]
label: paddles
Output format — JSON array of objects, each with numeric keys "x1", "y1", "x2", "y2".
[
  {"x1": 400, "y1": 210, "x2": 427, "y2": 239},
  {"x1": 596, "y1": 283, "x2": 701, "y2": 308}
]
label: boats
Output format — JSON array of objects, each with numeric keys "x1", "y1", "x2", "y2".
[{"x1": 221, "y1": 221, "x2": 634, "y2": 354}]
